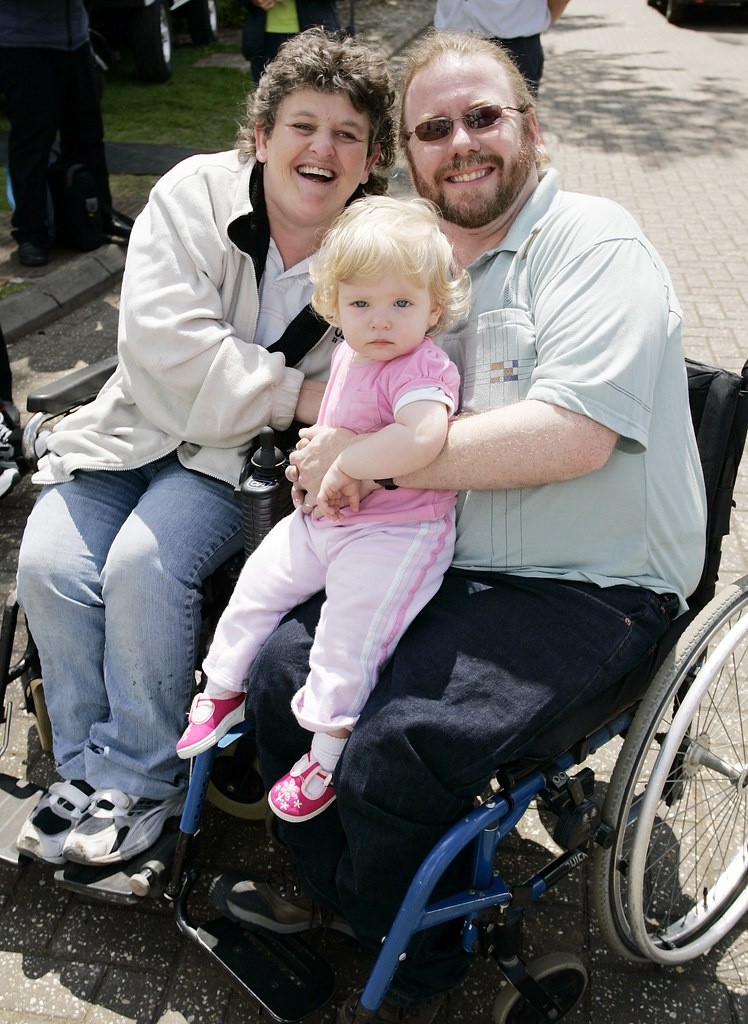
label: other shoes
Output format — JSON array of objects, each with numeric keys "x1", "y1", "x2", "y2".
[
  {"x1": 107, "y1": 210, "x2": 136, "y2": 243},
  {"x1": 17, "y1": 238, "x2": 48, "y2": 266},
  {"x1": 1, "y1": 400, "x2": 21, "y2": 430},
  {"x1": 268, "y1": 750, "x2": 337, "y2": 823},
  {"x1": 175, "y1": 692, "x2": 246, "y2": 759}
]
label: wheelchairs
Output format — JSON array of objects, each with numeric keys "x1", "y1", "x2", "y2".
[{"x1": 0, "y1": 353, "x2": 748, "y2": 1024}]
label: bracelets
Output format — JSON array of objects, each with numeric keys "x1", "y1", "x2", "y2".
[{"x1": 373, "y1": 478, "x2": 399, "y2": 490}]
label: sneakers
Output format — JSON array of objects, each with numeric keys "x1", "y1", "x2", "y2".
[
  {"x1": 16, "y1": 779, "x2": 99, "y2": 865},
  {"x1": 227, "y1": 860, "x2": 360, "y2": 940},
  {"x1": 372, "y1": 983, "x2": 448, "y2": 1024},
  {"x1": 61, "y1": 786, "x2": 190, "y2": 865}
]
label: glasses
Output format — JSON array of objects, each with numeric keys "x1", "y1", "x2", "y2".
[{"x1": 406, "y1": 103, "x2": 526, "y2": 142}]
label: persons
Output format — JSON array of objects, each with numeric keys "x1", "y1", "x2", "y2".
[
  {"x1": 206, "y1": 34, "x2": 708, "y2": 1023},
  {"x1": 433, "y1": 0, "x2": 570, "y2": 101},
  {"x1": 15, "y1": 25, "x2": 402, "y2": 867},
  {"x1": 175, "y1": 191, "x2": 473, "y2": 823},
  {"x1": 1, "y1": 0, "x2": 114, "y2": 268},
  {"x1": 233, "y1": 0, "x2": 342, "y2": 83}
]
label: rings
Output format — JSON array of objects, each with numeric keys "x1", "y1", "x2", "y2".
[{"x1": 304, "y1": 499, "x2": 314, "y2": 508}]
